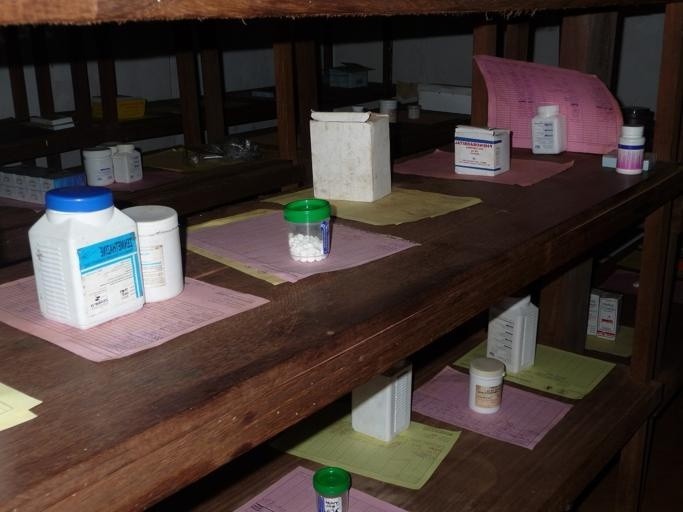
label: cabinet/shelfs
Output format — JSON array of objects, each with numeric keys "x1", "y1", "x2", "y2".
[
  {"x1": 0, "y1": 1, "x2": 683, "y2": 512},
  {"x1": 351, "y1": 357, "x2": 412, "y2": 443},
  {"x1": 313, "y1": 466, "x2": 352, "y2": 512},
  {"x1": 380, "y1": 100, "x2": 397, "y2": 123},
  {"x1": 27, "y1": 182, "x2": 184, "y2": 330},
  {"x1": 283, "y1": 198, "x2": 331, "y2": 263},
  {"x1": 352, "y1": 104, "x2": 364, "y2": 112},
  {"x1": 468, "y1": 357, "x2": 506, "y2": 414},
  {"x1": 407, "y1": 105, "x2": 421, "y2": 120},
  {"x1": 82, "y1": 136, "x2": 143, "y2": 186},
  {"x1": 0, "y1": 0, "x2": 683, "y2": 511},
  {"x1": 615, "y1": 125, "x2": 646, "y2": 175},
  {"x1": 486, "y1": 293, "x2": 539, "y2": 374},
  {"x1": 531, "y1": 104, "x2": 567, "y2": 155}
]
